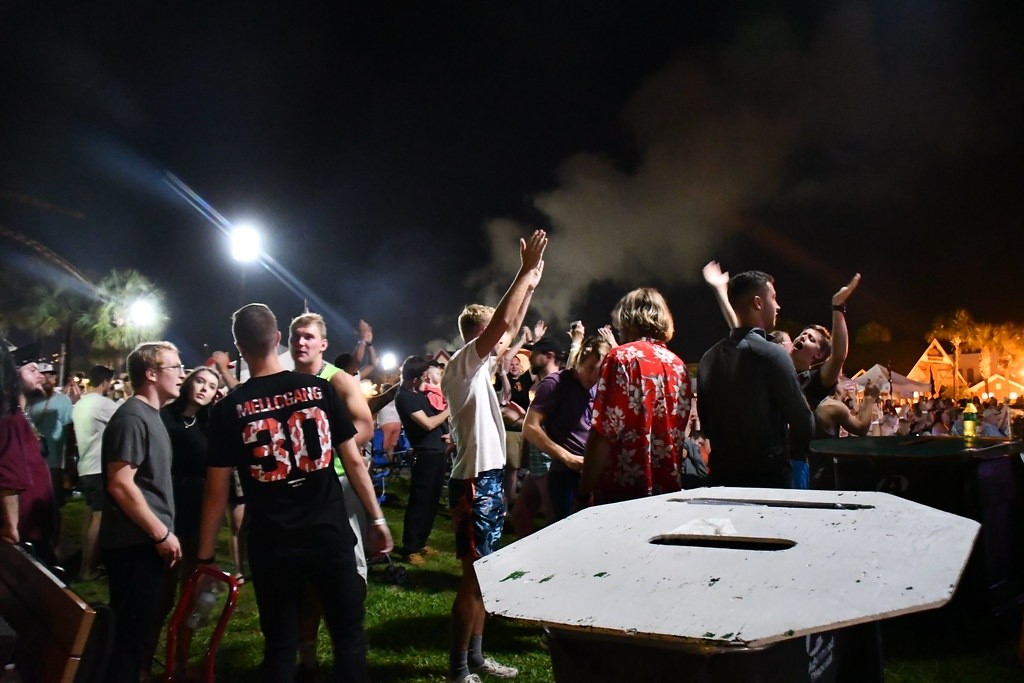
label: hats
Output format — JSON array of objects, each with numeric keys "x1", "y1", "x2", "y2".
[
  {"x1": 38, "y1": 362, "x2": 53, "y2": 372},
  {"x1": 521, "y1": 337, "x2": 564, "y2": 359},
  {"x1": 204, "y1": 356, "x2": 235, "y2": 369},
  {"x1": 430, "y1": 360, "x2": 445, "y2": 366}
]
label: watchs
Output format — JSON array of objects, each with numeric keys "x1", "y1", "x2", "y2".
[{"x1": 529, "y1": 339, "x2": 534, "y2": 343}]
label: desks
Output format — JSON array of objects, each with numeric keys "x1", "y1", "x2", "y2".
[
  {"x1": 473, "y1": 487, "x2": 982, "y2": 683},
  {"x1": 809, "y1": 435, "x2": 1022, "y2": 659}
]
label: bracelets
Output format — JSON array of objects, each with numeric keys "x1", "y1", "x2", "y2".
[
  {"x1": 71, "y1": 384, "x2": 73, "y2": 387},
  {"x1": 369, "y1": 518, "x2": 386, "y2": 527},
  {"x1": 832, "y1": 304, "x2": 846, "y2": 314},
  {"x1": 499, "y1": 369, "x2": 508, "y2": 376},
  {"x1": 197, "y1": 553, "x2": 216, "y2": 564},
  {"x1": 357, "y1": 340, "x2": 367, "y2": 345},
  {"x1": 153, "y1": 529, "x2": 169, "y2": 544},
  {"x1": 514, "y1": 417, "x2": 521, "y2": 423},
  {"x1": 367, "y1": 343, "x2": 372, "y2": 346}
]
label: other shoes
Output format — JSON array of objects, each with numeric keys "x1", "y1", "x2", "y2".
[
  {"x1": 416, "y1": 546, "x2": 439, "y2": 556},
  {"x1": 405, "y1": 552, "x2": 426, "y2": 565},
  {"x1": 517, "y1": 468, "x2": 530, "y2": 478},
  {"x1": 76, "y1": 569, "x2": 101, "y2": 581},
  {"x1": 73, "y1": 491, "x2": 82, "y2": 500}
]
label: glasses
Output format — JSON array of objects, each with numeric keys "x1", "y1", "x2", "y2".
[
  {"x1": 150, "y1": 364, "x2": 185, "y2": 371},
  {"x1": 366, "y1": 550, "x2": 390, "y2": 566}
]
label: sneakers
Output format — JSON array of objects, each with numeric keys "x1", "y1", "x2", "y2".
[
  {"x1": 470, "y1": 656, "x2": 519, "y2": 679},
  {"x1": 447, "y1": 673, "x2": 482, "y2": 683}
]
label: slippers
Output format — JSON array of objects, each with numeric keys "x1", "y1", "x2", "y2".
[{"x1": 236, "y1": 576, "x2": 246, "y2": 586}]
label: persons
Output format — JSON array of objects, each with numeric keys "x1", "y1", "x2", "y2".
[{"x1": 0, "y1": 229, "x2": 1024, "y2": 683}]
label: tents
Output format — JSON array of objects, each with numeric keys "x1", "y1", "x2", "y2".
[{"x1": 854, "y1": 363, "x2": 932, "y2": 404}]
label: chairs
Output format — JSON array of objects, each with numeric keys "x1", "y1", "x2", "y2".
[{"x1": 361, "y1": 426, "x2": 416, "y2": 506}]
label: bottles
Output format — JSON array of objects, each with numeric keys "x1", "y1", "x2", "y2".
[
  {"x1": 187, "y1": 583, "x2": 217, "y2": 630},
  {"x1": 58, "y1": 490, "x2": 91, "y2": 581},
  {"x1": 962, "y1": 399, "x2": 978, "y2": 437}
]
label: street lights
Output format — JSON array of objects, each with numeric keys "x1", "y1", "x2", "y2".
[{"x1": 56, "y1": 293, "x2": 154, "y2": 391}]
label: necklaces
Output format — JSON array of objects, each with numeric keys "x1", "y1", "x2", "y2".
[
  {"x1": 318, "y1": 364, "x2": 326, "y2": 374},
  {"x1": 29, "y1": 395, "x2": 50, "y2": 427},
  {"x1": 184, "y1": 416, "x2": 196, "y2": 428},
  {"x1": 24, "y1": 412, "x2": 44, "y2": 441}
]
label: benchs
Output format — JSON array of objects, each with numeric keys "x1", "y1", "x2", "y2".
[{"x1": 0, "y1": 534, "x2": 117, "y2": 683}]
